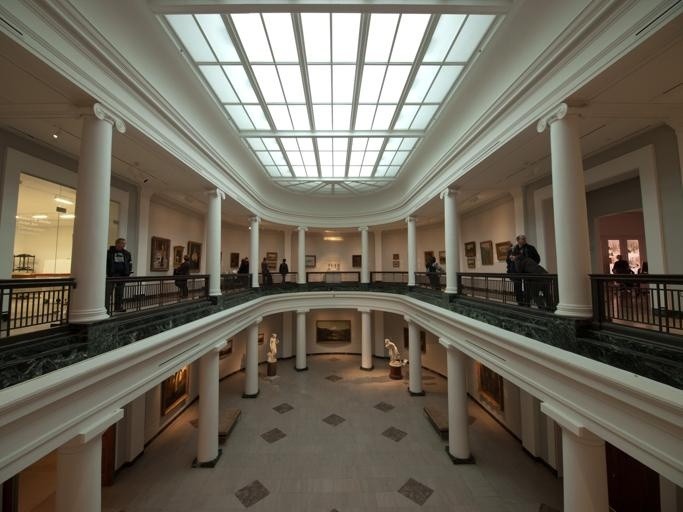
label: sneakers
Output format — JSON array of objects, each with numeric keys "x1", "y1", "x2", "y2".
[{"x1": 114, "y1": 307, "x2": 126, "y2": 313}]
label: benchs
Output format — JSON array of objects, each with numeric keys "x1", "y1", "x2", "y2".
[
  {"x1": 214, "y1": 408, "x2": 241, "y2": 445},
  {"x1": 424, "y1": 406, "x2": 451, "y2": 439}
]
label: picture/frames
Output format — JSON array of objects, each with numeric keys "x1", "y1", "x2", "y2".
[
  {"x1": 404, "y1": 327, "x2": 426, "y2": 353},
  {"x1": 316, "y1": 320, "x2": 351, "y2": 344},
  {"x1": 160, "y1": 366, "x2": 191, "y2": 417},
  {"x1": 478, "y1": 363, "x2": 504, "y2": 412},
  {"x1": 258, "y1": 334, "x2": 264, "y2": 345},
  {"x1": 219, "y1": 340, "x2": 232, "y2": 360}
]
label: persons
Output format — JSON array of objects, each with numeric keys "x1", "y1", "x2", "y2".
[
  {"x1": 261, "y1": 257, "x2": 272, "y2": 285},
  {"x1": 279, "y1": 258, "x2": 288, "y2": 283},
  {"x1": 105, "y1": 238, "x2": 132, "y2": 312},
  {"x1": 238, "y1": 257, "x2": 249, "y2": 274},
  {"x1": 384, "y1": 338, "x2": 400, "y2": 364},
  {"x1": 269, "y1": 333, "x2": 279, "y2": 356},
  {"x1": 177, "y1": 254, "x2": 191, "y2": 298},
  {"x1": 508, "y1": 250, "x2": 553, "y2": 309},
  {"x1": 612, "y1": 254, "x2": 629, "y2": 274},
  {"x1": 506, "y1": 234, "x2": 541, "y2": 307},
  {"x1": 426, "y1": 255, "x2": 444, "y2": 290}
]
